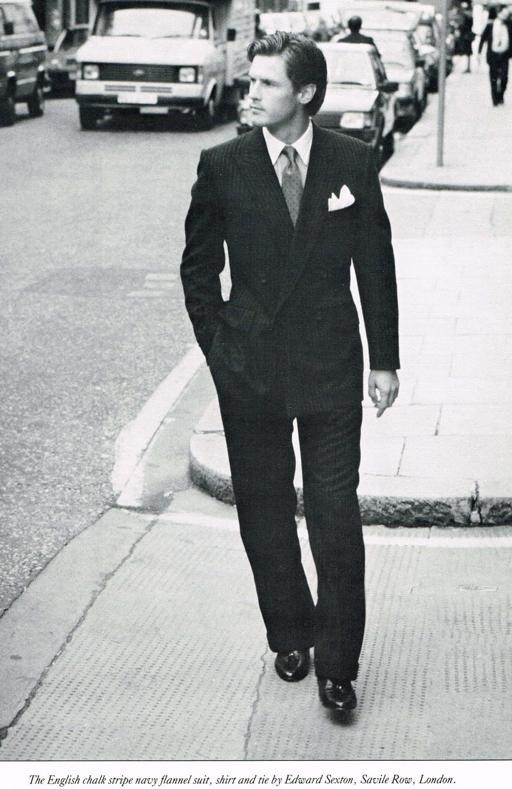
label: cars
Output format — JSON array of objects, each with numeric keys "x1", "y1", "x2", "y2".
[
  {"x1": 0, "y1": 0, "x2": 91, "y2": 128},
  {"x1": 255, "y1": 0, "x2": 462, "y2": 173}
]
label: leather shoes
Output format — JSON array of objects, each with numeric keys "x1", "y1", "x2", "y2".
[
  {"x1": 275, "y1": 648, "x2": 311, "y2": 683},
  {"x1": 317, "y1": 675, "x2": 358, "y2": 710}
]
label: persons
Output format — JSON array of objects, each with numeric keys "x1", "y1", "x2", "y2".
[
  {"x1": 338, "y1": 17, "x2": 375, "y2": 44},
  {"x1": 457, "y1": 5, "x2": 512, "y2": 105},
  {"x1": 180, "y1": 31, "x2": 399, "y2": 714}
]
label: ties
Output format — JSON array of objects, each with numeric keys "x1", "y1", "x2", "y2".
[{"x1": 280, "y1": 144, "x2": 304, "y2": 229}]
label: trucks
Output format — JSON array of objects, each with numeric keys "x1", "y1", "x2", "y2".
[{"x1": 72, "y1": 0, "x2": 259, "y2": 132}]
